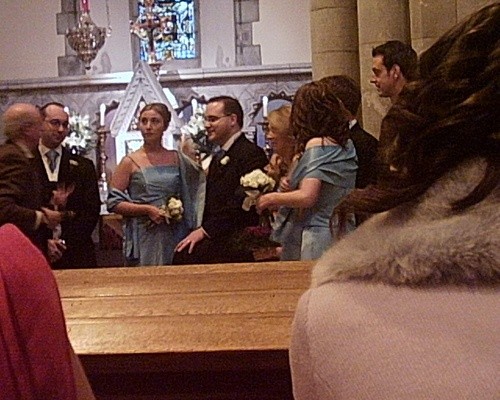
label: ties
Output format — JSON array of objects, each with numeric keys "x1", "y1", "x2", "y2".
[{"x1": 45, "y1": 150, "x2": 60, "y2": 173}]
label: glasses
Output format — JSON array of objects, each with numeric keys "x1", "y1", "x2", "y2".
[
  {"x1": 45, "y1": 120, "x2": 70, "y2": 129},
  {"x1": 205, "y1": 114, "x2": 232, "y2": 122}
]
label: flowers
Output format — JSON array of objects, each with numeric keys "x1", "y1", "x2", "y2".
[
  {"x1": 68, "y1": 158, "x2": 79, "y2": 176},
  {"x1": 219, "y1": 156, "x2": 231, "y2": 170},
  {"x1": 143, "y1": 196, "x2": 185, "y2": 232},
  {"x1": 61, "y1": 108, "x2": 97, "y2": 155},
  {"x1": 239, "y1": 169, "x2": 276, "y2": 223},
  {"x1": 179, "y1": 103, "x2": 208, "y2": 139}
]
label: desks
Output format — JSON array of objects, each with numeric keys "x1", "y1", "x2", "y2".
[{"x1": 50, "y1": 260, "x2": 316, "y2": 376}]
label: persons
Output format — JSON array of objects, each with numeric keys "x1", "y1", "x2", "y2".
[
  {"x1": 288, "y1": 0, "x2": 500, "y2": 399},
  {"x1": 0, "y1": 39, "x2": 418, "y2": 400}
]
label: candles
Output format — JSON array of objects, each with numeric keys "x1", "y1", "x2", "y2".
[
  {"x1": 262, "y1": 96, "x2": 269, "y2": 117},
  {"x1": 99, "y1": 103, "x2": 106, "y2": 126}
]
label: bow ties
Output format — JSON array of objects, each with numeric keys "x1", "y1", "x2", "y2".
[{"x1": 212, "y1": 150, "x2": 226, "y2": 160}]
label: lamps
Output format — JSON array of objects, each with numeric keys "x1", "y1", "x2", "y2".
[{"x1": 65, "y1": 0, "x2": 106, "y2": 70}]
